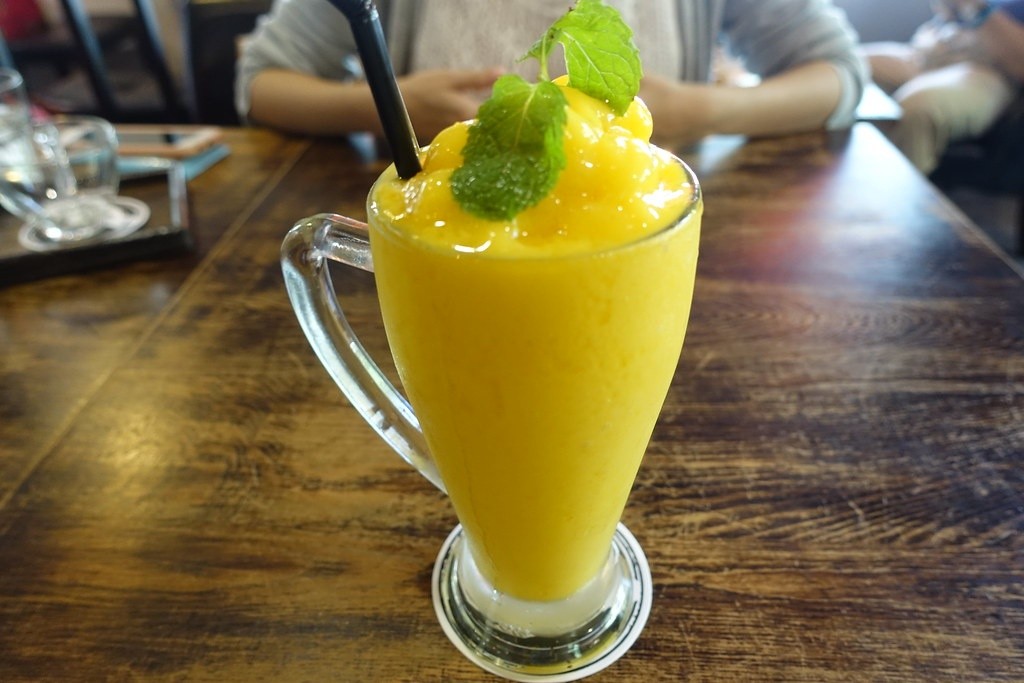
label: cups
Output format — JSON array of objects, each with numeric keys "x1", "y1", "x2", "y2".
[{"x1": 0, "y1": 118, "x2": 151, "y2": 250}]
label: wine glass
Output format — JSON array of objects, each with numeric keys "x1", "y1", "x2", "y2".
[{"x1": 280, "y1": 142, "x2": 702, "y2": 683}]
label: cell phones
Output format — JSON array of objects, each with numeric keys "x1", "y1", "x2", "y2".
[{"x1": 115, "y1": 132, "x2": 223, "y2": 156}]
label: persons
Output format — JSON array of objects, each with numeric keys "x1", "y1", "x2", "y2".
[
  {"x1": 855, "y1": 1, "x2": 1024, "y2": 174},
  {"x1": 233, "y1": 0, "x2": 867, "y2": 144}
]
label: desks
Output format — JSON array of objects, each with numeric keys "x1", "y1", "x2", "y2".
[{"x1": 0, "y1": 123, "x2": 1024, "y2": 683}]
